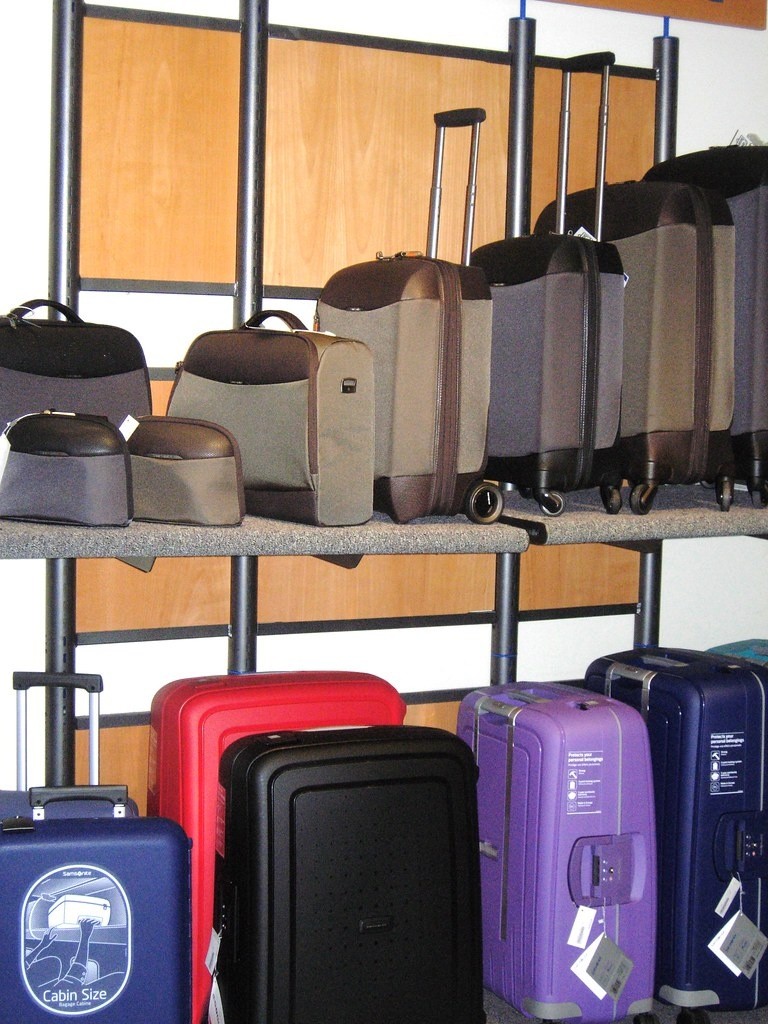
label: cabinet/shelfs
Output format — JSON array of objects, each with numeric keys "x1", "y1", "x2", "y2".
[{"x1": 0, "y1": 2, "x2": 768, "y2": 1024}]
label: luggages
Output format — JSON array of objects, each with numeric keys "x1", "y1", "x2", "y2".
[
  {"x1": 0, "y1": 638, "x2": 768, "y2": 1024},
  {"x1": 317, "y1": 52, "x2": 768, "y2": 524}
]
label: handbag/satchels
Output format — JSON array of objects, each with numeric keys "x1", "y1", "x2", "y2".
[
  {"x1": 126, "y1": 416, "x2": 245, "y2": 529},
  {"x1": 2, "y1": 409, "x2": 133, "y2": 530},
  {"x1": 166, "y1": 310, "x2": 375, "y2": 526},
  {"x1": 0, "y1": 299, "x2": 152, "y2": 439}
]
